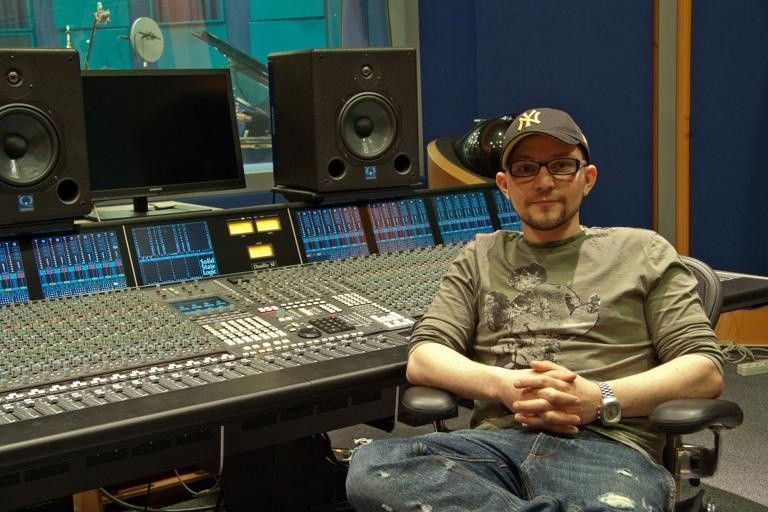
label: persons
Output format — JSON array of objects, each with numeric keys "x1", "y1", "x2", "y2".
[{"x1": 341, "y1": 107, "x2": 726, "y2": 512}]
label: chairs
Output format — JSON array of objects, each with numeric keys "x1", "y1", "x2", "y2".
[{"x1": 403, "y1": 253, "x2": 744, "y2": 512}]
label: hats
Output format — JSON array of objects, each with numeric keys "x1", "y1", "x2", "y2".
[{"x1": 500, "y1": 107, "x2": 590, "y2": 170}]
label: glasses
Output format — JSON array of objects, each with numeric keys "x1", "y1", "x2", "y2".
[{"x1": 507, "y1": 157, "x2": 587, "y2": 177}]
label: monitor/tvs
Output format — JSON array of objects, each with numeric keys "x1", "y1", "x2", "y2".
[{"x1": 80, "y1": 67, "x2": 248, "y2": 221}]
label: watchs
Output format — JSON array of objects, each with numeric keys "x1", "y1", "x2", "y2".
[{"x1": 596, "y1": 380, "x2": 622, "y2": 428}]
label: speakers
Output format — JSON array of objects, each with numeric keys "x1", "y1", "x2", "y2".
[
  {"x1": 0, "y1": 47, "x2": 93, "y2": 237},
  {"x1": 267, "y1": 46, "x2": 421, "y2": 194}
]
